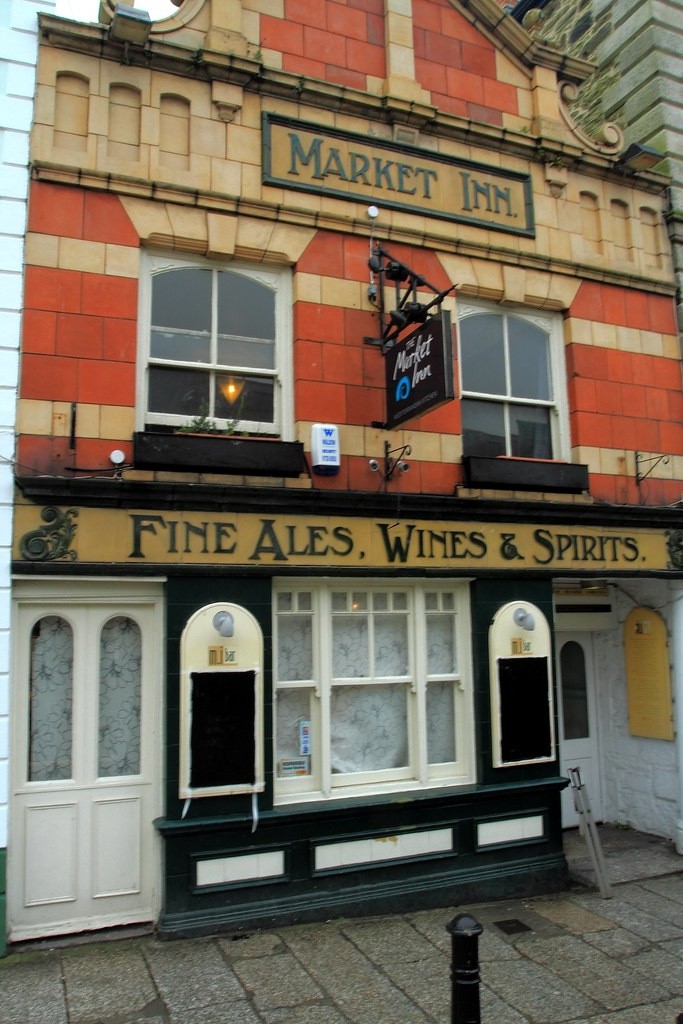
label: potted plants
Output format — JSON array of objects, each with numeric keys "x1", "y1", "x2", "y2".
[{"x1": 173, "y1": 402, "x2": 282, "y2": 442}]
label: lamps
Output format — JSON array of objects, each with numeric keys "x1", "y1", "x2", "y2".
[
  {"x1": 109, "y1": 3, "x2": 153, "y2": 48},
  {"x1": 515, "y1": 609, "x2": 535, "y2": 631},
  {"x1": 218, "y1": 378, "x2": 245, "y2": 405},
  {"x1": 216, "y1": 611, "x2": 233, "y2": 636},
  {"x1": 611, "y1": 142, "x2": 666, "y2": 176}
]
